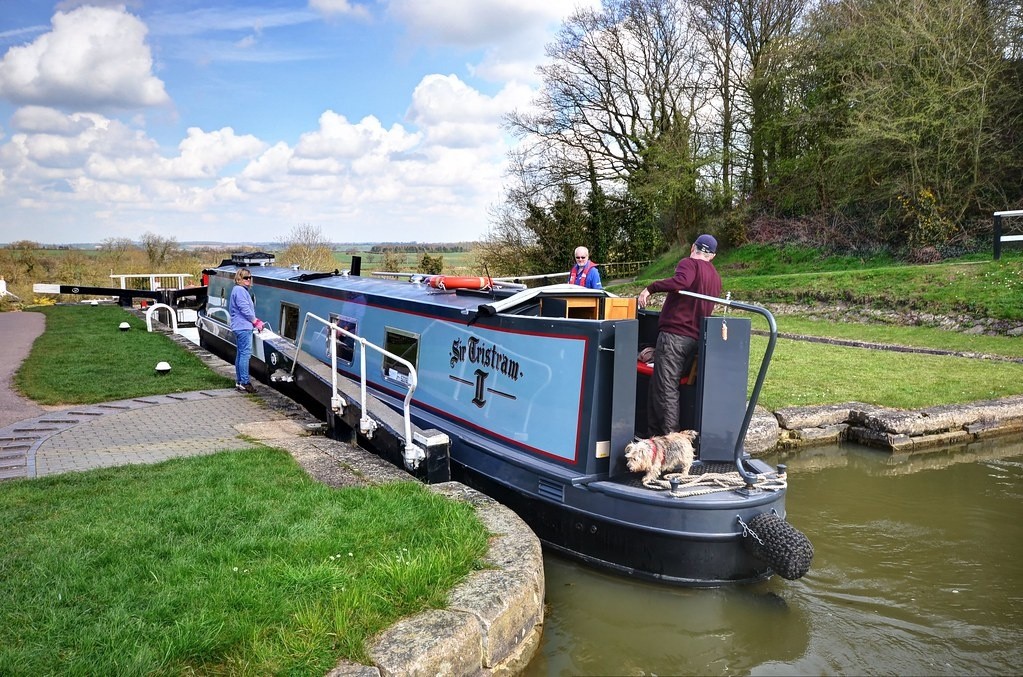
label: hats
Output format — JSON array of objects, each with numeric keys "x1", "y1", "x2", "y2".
[{"x1": 695, "y1": 235, "x2": 717, "y2": 253}]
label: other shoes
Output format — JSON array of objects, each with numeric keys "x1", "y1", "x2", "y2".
[{"x1": 236, "y1": 382, "x2": 259, "y2": 393}]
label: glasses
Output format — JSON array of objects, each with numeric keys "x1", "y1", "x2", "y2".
[
  {"x1": 243, "y1": 275, "x2": 251, "y2": 279},
  {"x1": 576, "y1": 256, "x2": 585, "y2": 258}
]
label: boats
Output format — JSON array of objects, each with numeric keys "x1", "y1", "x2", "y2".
[{"x1": 195, "y1": 250, "x2": 817, "y2": 589}]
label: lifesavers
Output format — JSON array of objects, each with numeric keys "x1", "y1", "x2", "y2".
[{"x1": 429, "y1": 274, "x2": 494, "y2": 291}]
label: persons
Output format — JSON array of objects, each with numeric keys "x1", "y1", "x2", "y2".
[
  {"x1": 0, "y1": 274, "x2": 6, "y2": 295},
  {"x1": 228, "y1": 268, "x2": 265, "y2": 392},
  {"x1": 567, "y1": 245, "x2": 602, "y2": 289},
  {"x1": 637, "y1": 233, "x2": 723, "y2": 434}
]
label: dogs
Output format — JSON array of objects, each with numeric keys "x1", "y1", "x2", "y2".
[{"x1": 624, "y1": 429, "x2": 699, "y2": 484}]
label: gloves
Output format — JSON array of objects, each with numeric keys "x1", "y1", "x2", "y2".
[{"x1": 252, "y1": 318, "x2": 264, "y2": 332}]
